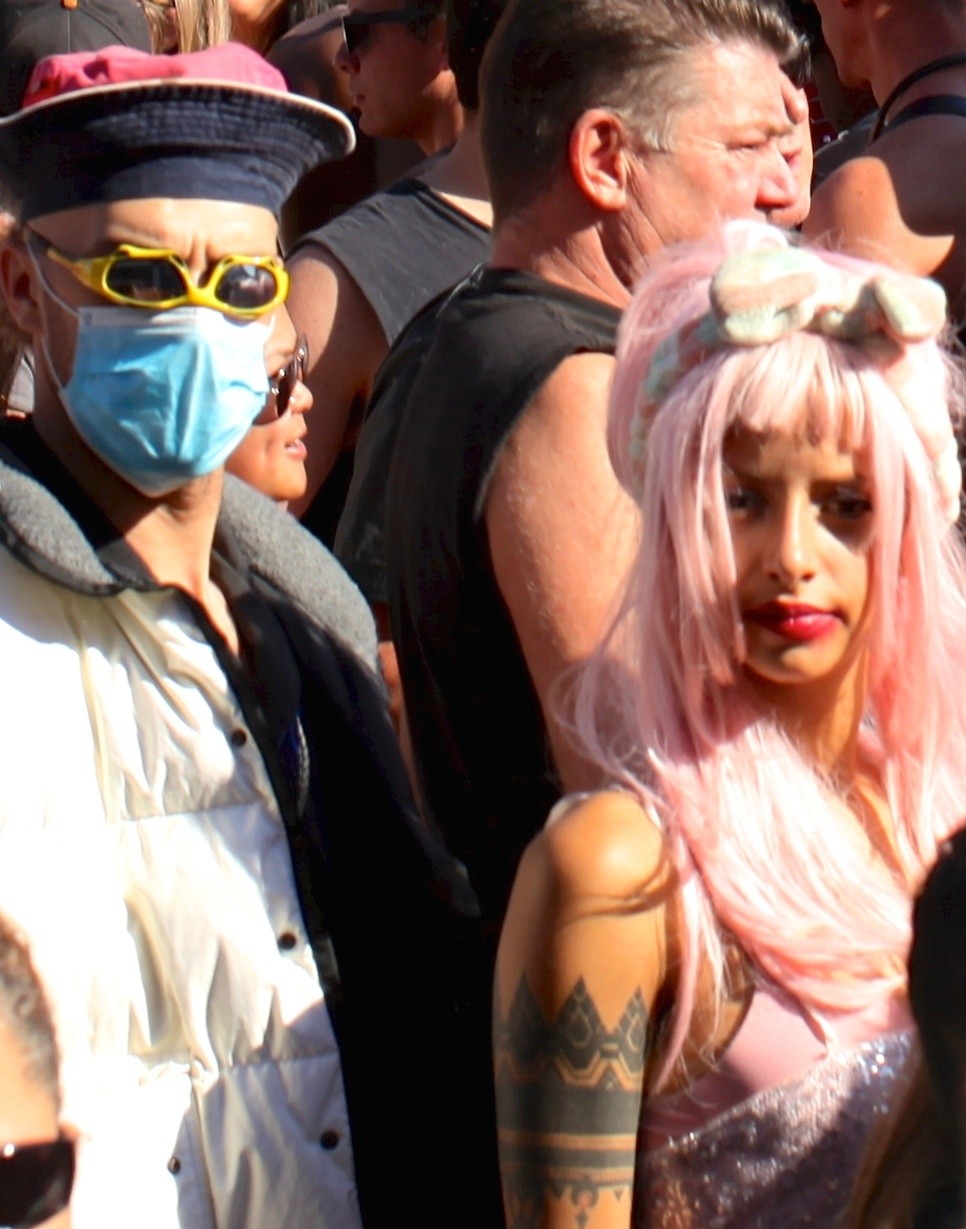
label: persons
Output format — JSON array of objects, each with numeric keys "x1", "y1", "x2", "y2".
[
  {"x1": 0, "y1": 0, "x2": 963, "y2": 906},
  {"x1": 0, "y1": 34, "x2": 482, "y2": 1229},
  {"x1": 0, "y1": 917, "x2": 80, "y2": 1229},
  {"x1": 831, "y1": 818, "x2": 966, "y2": 1226},
  {"x1": 490, "y1": 219, "x2": 963, "y2": 1229}
]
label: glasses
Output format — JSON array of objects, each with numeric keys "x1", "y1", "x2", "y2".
[
  {"x1": 342, "y1": 11, "x2": 412, "y2": 48},
  {"x1": 257, "y1": 335, "x2": 312, "y2": 424},
  {"x1": 25, "y1": 234, "x2": 287, "y2": 318}
]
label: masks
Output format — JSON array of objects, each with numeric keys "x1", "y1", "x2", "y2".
[{"x1": 35, "y1": 269, "x2": 272, "y2": 498}]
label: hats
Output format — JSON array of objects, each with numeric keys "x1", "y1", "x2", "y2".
[
  {"x1": 1, "y1": 43, "x2": 358, "y2": 221},
  {"x1": 9, "y1": 2, "x2": 149, "y2": 97}
]
